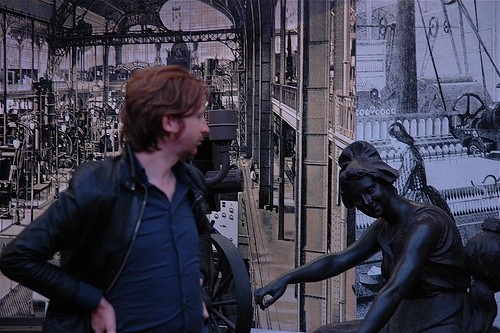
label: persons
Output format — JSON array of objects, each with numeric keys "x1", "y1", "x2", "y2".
[
  {"x1": 254, "y1": 140, "x2": 471, "y2": 333},
  {"x1": 0, "y1": 64, "x2": 211, "y2": 333}
]
